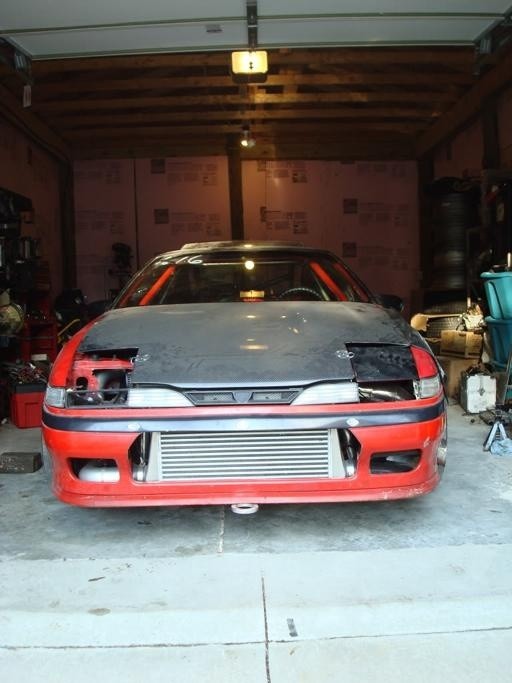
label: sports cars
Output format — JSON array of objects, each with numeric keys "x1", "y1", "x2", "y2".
[{"x1": 38, "y1": 236, "x2": 453, "y2": 516}]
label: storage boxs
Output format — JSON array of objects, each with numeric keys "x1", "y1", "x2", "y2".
[
  {"x1": 441, "y1": 329, "x2": 483, "y2": 359},
  {"x1": 484, "y1": 315, "x2": 512, "y2": 366},
  {"x1": 459, "y1": 371, "x2": 497, "y2": 414},
  {"x1": 435, "y1": 355, "x2": 481, "y2": 398},
  {"x1": 481, "y1": 270, "x2": 512, "y2": 320},
  {"x1": 9, "y1": 383, "x2": 45, "y2": 429}
]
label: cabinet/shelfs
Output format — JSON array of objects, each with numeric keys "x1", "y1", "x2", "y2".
[{"x1": 0, "y1": 316, "x2": 58, "y2": 364}]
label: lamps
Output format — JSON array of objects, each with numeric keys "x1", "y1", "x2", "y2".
[
  {"x1": 240, "y1": 124, "x2": 257, "y2": 148},
  {"x1": 231, "y1": 5, "x2": 268, "y2": 74}
]
